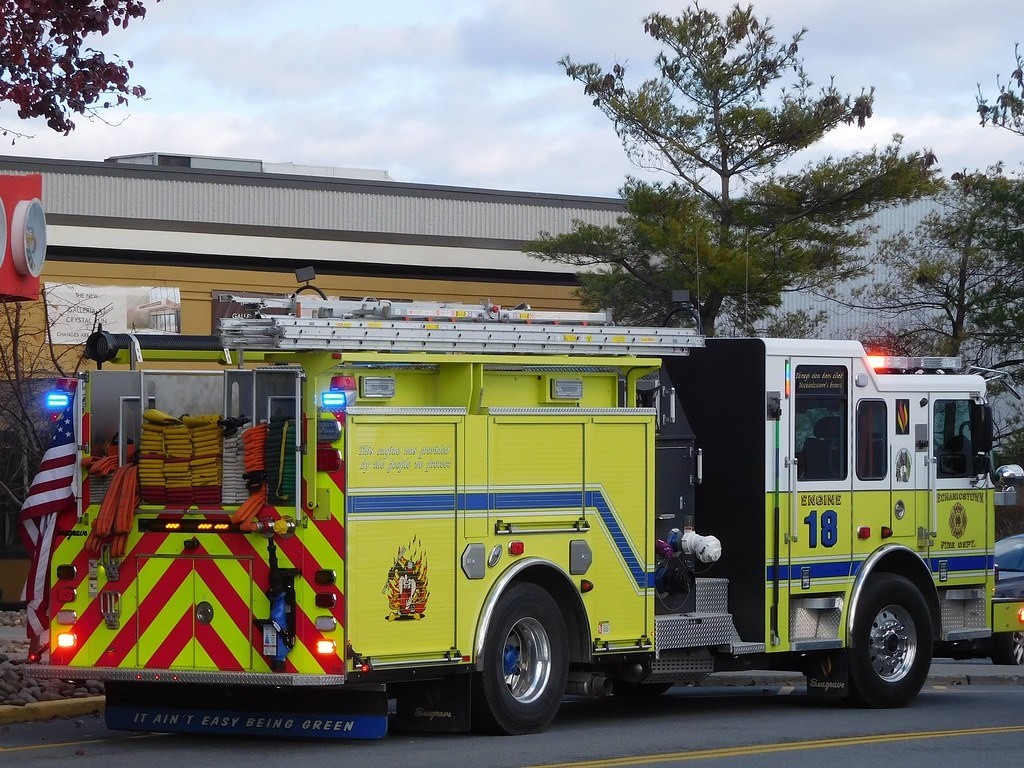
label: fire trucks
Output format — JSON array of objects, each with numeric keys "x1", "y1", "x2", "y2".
[{"x1": 17, "y1": 266, "x2": 1023, "y2": 741}]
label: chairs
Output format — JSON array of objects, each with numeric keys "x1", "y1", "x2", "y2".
[{"x1": 802, "y1": 417, "x2": 842, "y2": 480}]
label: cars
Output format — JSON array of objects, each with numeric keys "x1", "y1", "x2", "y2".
[{"x1": 993, "y1": 534, "x2": 1024, "y2": 666}]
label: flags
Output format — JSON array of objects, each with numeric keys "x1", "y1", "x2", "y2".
[{"x1": 17, "y1": 384, "x2": 80, "y2": 639}]
